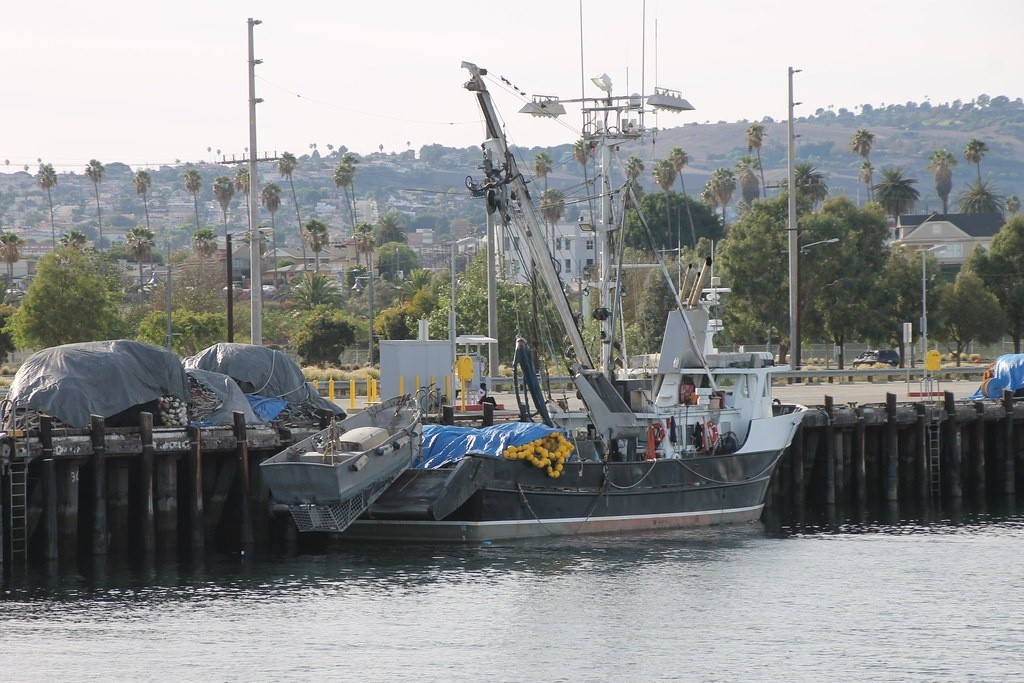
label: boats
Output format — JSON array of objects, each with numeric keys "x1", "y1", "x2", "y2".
[{"x1": 260, "y1": 392, "x2": 425, "y2": 508}]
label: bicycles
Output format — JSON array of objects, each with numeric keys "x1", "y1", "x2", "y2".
[{"x1": 418, "y1": 383, "x2": 450, "y2": 414}]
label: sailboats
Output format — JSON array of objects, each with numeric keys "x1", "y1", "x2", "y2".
[{"x1": 287, "y1": 0, "x2": 807, "y2": 544}]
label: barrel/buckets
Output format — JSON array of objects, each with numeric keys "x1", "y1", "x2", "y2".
[{"x1": 709, "y1": 396, "x2": 721, "y2": 409}]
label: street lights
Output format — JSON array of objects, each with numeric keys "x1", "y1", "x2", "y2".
[
  {"x1": 148, "y1": 270, "x2": 172, "y2": 352},
  {"x1": 787, "y1": 237, "x2": 840, "y2": 383},
  {"x1": 350, "y1": 276, "x2": 374, "y2": 371},
  {"x1": 919, "y1": 244, "x2": 947, "y2": 368}
]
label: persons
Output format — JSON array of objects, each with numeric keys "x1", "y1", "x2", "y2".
[{"x1": 477, "y1": 383, "x2": 498, "y2": 409}]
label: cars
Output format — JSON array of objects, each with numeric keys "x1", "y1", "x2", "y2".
[{"x1": 223, "y1": 284, "x2": 276, "y2": 295}]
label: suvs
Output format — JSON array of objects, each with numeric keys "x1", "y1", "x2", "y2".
[{"x1": 852, "y1": 348, "x2": 899, "y2": 368}]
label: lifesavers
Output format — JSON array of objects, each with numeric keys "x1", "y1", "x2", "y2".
[
  {"x1": 699, "y1": 422, "x2": 719, "y2": 448},
  {"x1": 647, "y1": 424, "x2": 665, "y2": 442}
]
